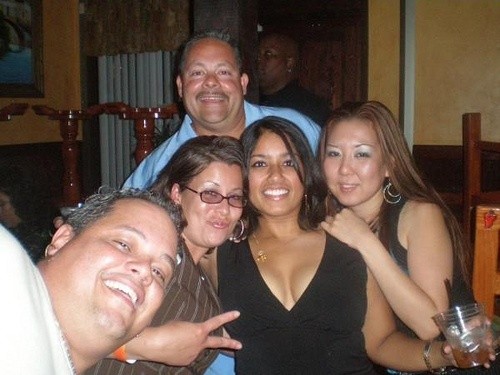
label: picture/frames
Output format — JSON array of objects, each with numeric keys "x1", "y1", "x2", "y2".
[{"x1": 0, "y1": 0, "x2": 45, "y2": 98}]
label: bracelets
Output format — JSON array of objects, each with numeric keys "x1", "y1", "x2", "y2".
[
  {"x1": 114, "y1": 345, "x2": 136, "y2": 364},
  {"x1": 423, "y1": 342, "x2": 447, "y2": 375}
]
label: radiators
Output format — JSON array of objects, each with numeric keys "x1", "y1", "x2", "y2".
[{"x1": 99, "y1": 51, "x2": 186, "y2": 191}]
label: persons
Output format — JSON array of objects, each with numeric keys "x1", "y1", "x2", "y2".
[
  {"x1": 316, "y1": 102, "x2": 479, "y2": 375},
  {"x1": 123, "y1": 30, "x2": 323, "y2": 197},
  {"x1": 0, "y1": 174, "x2": 49, "y2": 265},
  {"x1": 83, "y1": 135, "x2": 246, "y2": 375},
  {"x1": 199, "y1": 116, "x2": 496, "y2": 375},
  {"x1": 247, "y1": 33, "x2": 331, "y2": 128},
  {"x1": 0, "y1": 185, "x2": 188, "y2": 375}
]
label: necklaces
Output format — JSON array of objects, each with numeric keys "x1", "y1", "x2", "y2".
[{"x1": 252, "y1": 230, "x2": 267, "y2": 264}]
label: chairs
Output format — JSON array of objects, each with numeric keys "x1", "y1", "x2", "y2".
[{"x1": 464, "y1": 113, "x2": 500, "y2": 318}]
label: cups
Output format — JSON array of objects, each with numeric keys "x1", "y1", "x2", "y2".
[{"x1": 431, "y1": 302, "x2": 490, "y2": 368}]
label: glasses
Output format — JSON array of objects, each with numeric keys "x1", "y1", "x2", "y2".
[{"x1": 180, "y1": 185, "x2": 248, "y2": 208}]
